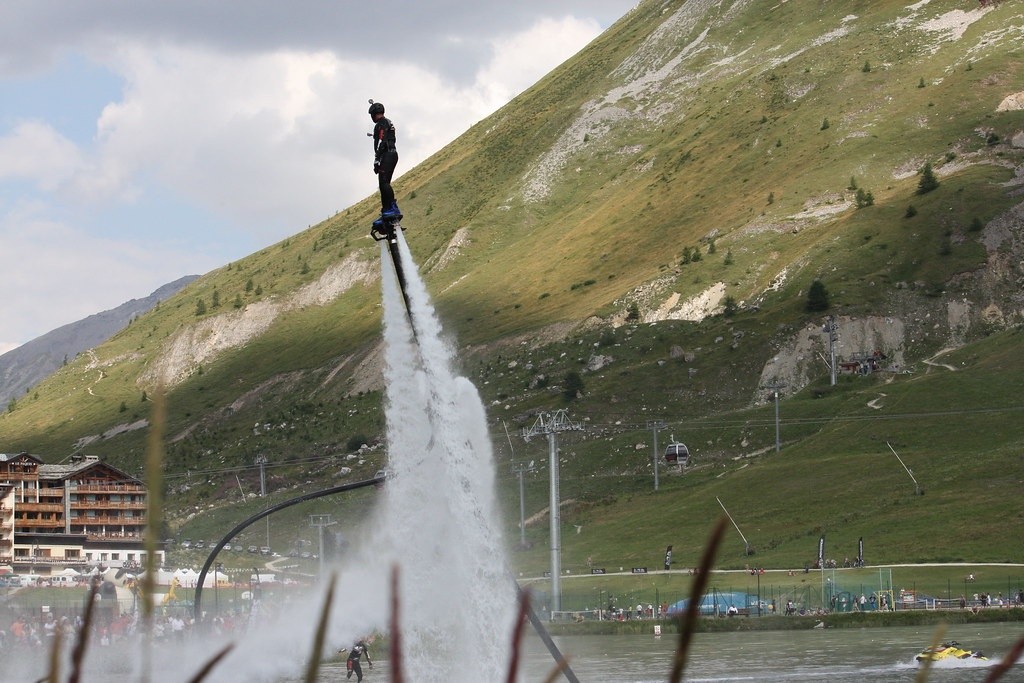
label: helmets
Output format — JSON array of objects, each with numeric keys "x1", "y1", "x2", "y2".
[{"x1": 369, "y1": 103, "x2": 384, "y2": 114}]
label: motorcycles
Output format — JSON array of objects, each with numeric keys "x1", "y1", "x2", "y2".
[{"x1": 915, "y1": 640, "x2": 990, "y2": 665}]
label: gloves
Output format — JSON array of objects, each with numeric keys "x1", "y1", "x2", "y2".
[{"x1": 374, "y1": 158, "x2": 380, "y2": 174}]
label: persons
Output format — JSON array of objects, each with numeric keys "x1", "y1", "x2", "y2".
[
  {"x1": 960, "y1": 573, "x2": 1024, "y2": 609},
  {"x1": 369, "y1": 103, "x2": 400, "y2": 226},
  {"x1": 0, "y1": 608, "x2": 246, "y2": 656},
  {"x1": 593, "y1": 555, "x2": 889, "y2": 622},
  {"x1": 346, "y1": 636, "x2": 374, "y2": 683}
]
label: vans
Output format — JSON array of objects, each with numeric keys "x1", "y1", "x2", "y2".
[{"x1": 1, "y1": 573, "x2": 105, "y2": 590}]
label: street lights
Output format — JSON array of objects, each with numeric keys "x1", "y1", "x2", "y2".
[{"x1": 215, "y1": 562, "x2": 223, "y2": 616}]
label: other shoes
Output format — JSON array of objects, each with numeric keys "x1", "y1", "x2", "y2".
[
  {"x1": 373, "y1": 218, "x2": 382, "y2": 226},
  {"x1": 382, "y1": 199, "x2": 400, "y2": 217}
]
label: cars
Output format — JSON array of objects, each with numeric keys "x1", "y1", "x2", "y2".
[
  {"x1": 165, "y1": 538, "x2": 270, "y2": 556},
  {"x1": 666, "y1": 591, "x2": 765, "y2": 616}
]
